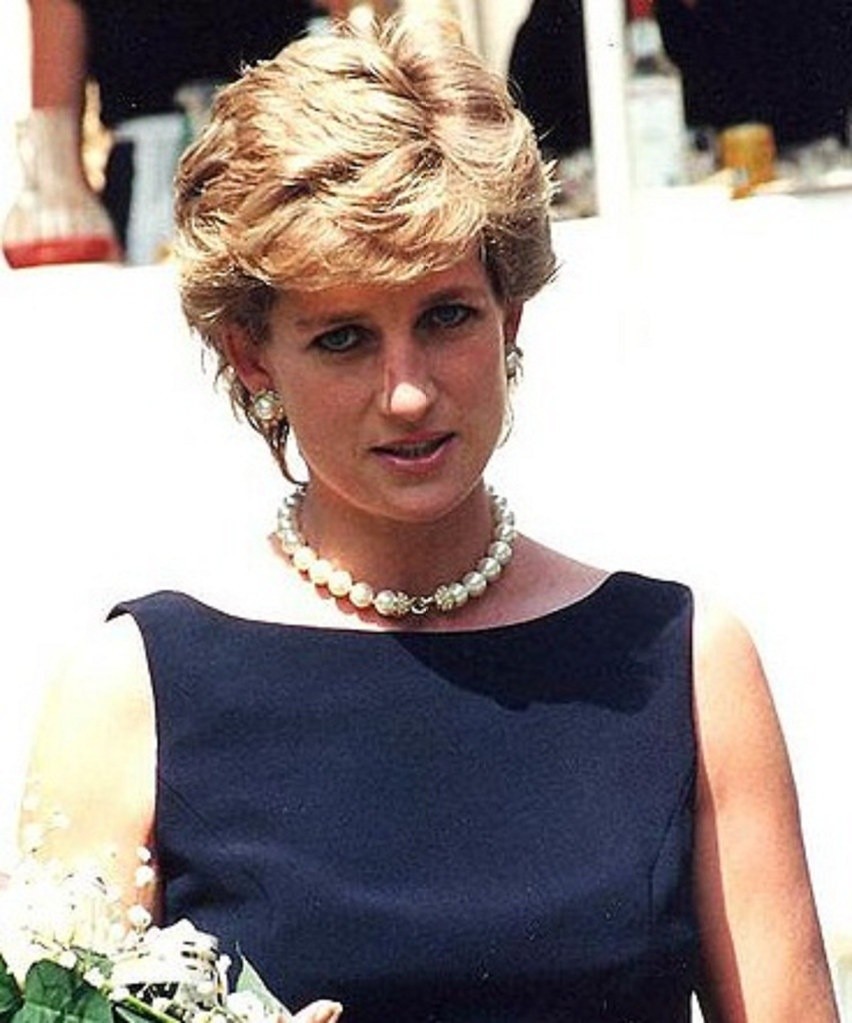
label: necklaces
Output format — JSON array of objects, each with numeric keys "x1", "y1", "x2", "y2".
[{"x1": 273, "y1": 482, "x2": 516, "y2": 614}]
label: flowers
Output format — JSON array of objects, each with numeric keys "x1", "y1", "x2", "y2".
[{"x1": 0, "y1": 783, "x2": 297, "y2": 1023}]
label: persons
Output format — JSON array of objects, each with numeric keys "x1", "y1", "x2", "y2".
[
  {"x1": 28, "y1": 0, "x2": 852, "y2": 259},
  {"x1": 21, "y1": 13, "x2": 841, "y2": 1023}
]
